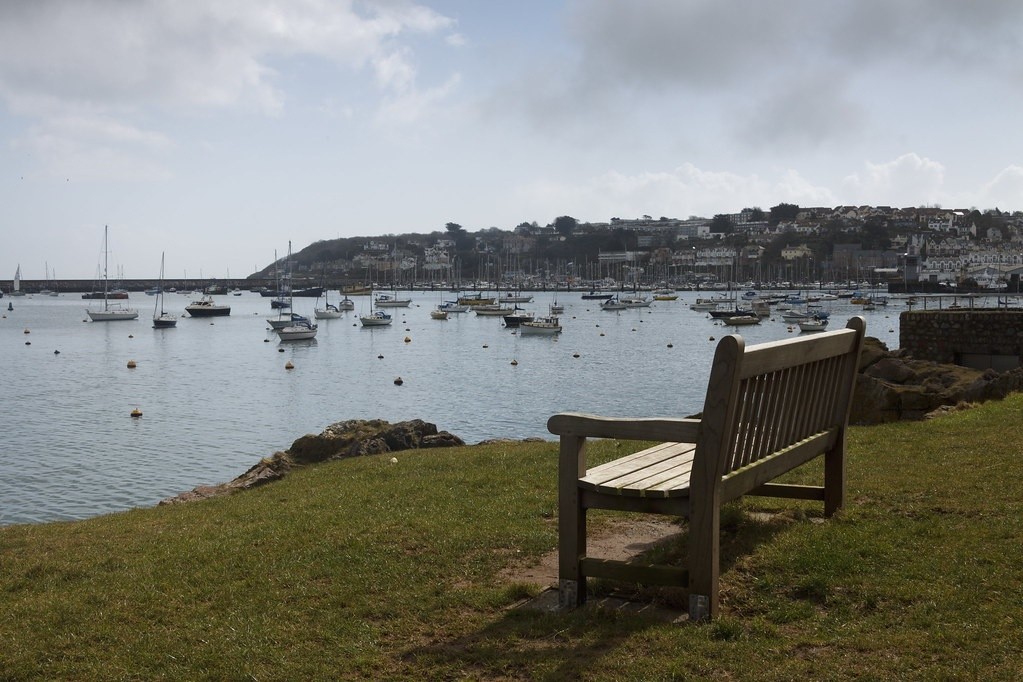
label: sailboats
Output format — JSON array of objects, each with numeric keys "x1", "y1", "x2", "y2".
[
  {"x1": 85, "y1": 225, "x2": 139, "y2": 322},
  {"x1": 50, "y1": 268, "x2": 60, "y2": 296},
  {"x1": 267, "y1": 235, "x2": 1023, "y2": 341},
  {"x1": 8, "y1": 264, "x2": 26, "y2": 296},
  {"x1": 40, "y1": 260, "x2": 52, "y2": 295},
  {"x1": 270, "y1": 249, "x2": 292, "y2": 309},
  {"x1": 177, "y1": 269, "x2": 192, "y2": 294},
  {"x1": 81, "y1": 264, "x2": 129, "y2": 299},
  {"x1": 250, "y1": 265, "x2": 260, "y2": 293},
  {"x1": 153, "y1": 252, "x2": 177, "y2": 328}
]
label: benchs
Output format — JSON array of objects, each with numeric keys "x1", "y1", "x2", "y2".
[{"x1": 546, "y1": 316, "x2": 866, "y2": 620}]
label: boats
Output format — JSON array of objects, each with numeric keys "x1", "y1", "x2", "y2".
[
  {"x1": 233, "y1": 288, "x2": 242, "y2": 296},
  {"x1": 260, "y1": 285, "x2": 323, "y2": 297},
  {"x1": 0, "y1": 290, "x2": 3, "y2": 298},
  {"x1": 203, "y1": 283, "x2": 227, "y2": 295},
  {"x1": 185, "y1": 295, "x2": 231, "y2": 317},
  {"x1": 145, "y1": 287, "x2": 163, "y2": 296},
  {"x1": 165, "y1": 287, "x2": 176, "y2": 292}
]
